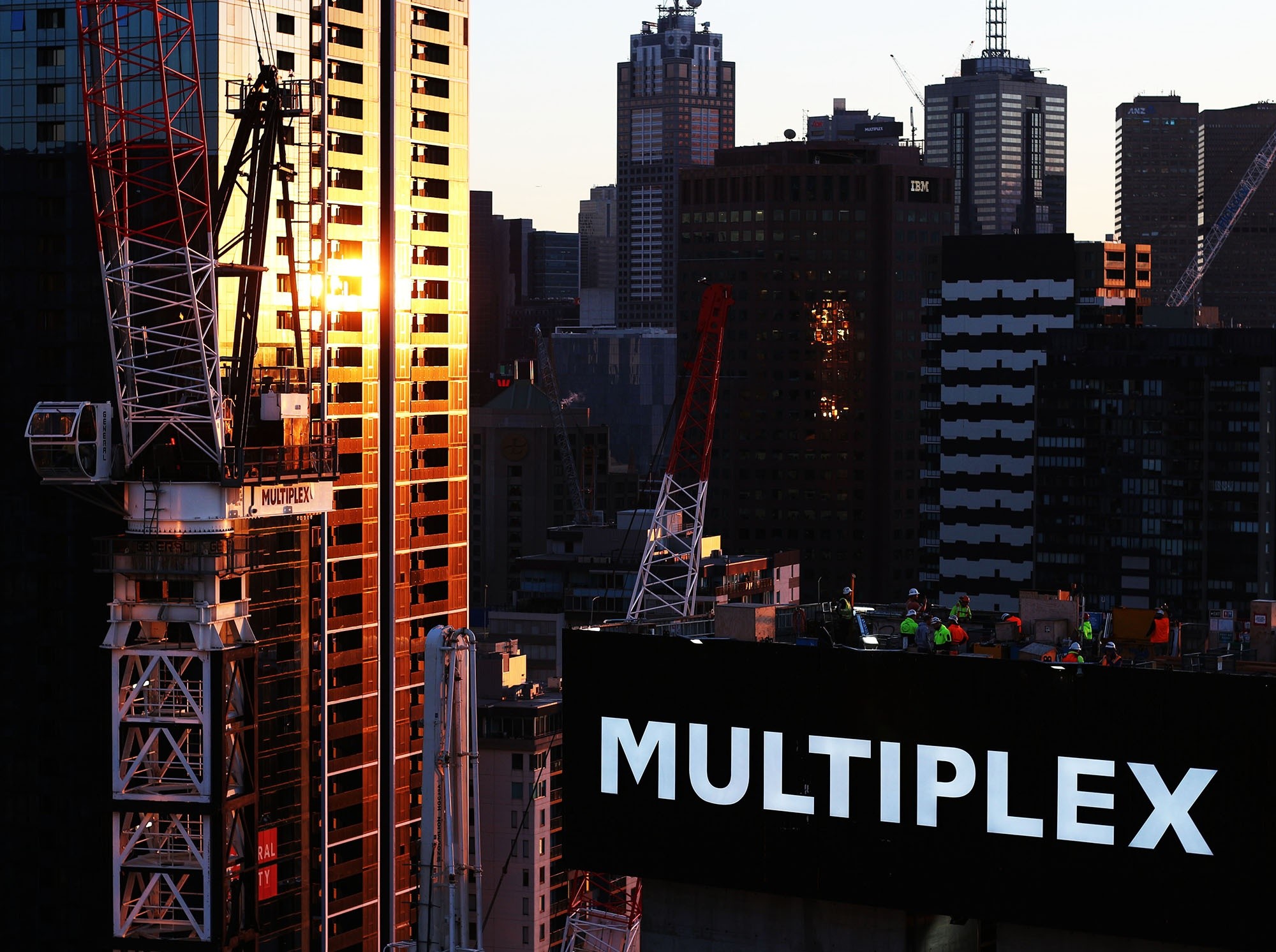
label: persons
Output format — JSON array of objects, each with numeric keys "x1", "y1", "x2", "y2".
[
  {"x1": 905, "y1": 588, "x2": 933, "y2": 621},
  {"x1": 1101, "y1": 641, "x2": 1123, "y2": 667},
  {"x1": 1000, "y1": 613, "x2": 1023, "y2": 642},
  {"x1": 836, "y1": 586, "x2": 853, "y2": 645},
  {"x1": 950, "y1": 594, "x2": 972, "y2": 623},
  {"x1": 1062, "y1": 641, "x2": 1085, "y2": 662},
  {"x1": 1072, "y1": 613, "x2": 1093, "y2": 662},
  {"x1": 1144, "y1": 609, "x2": 1170, "y2": 657},
  {"x1": 914, "y1": 613, "x2": 969, "y2": 654},
  {"x1": 899, "y1": 608, "x2": 919, "y2": 650}
]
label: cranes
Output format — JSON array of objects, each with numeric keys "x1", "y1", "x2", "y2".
[
  {"x1": 1166, "y1": 129, "x2": 1276, "y2": 309},
  {"x1": 23, "y1": 0, "x2": 338, "y2": 952},
  {"x1": 533, "y1": 323, "x2": 596, "y2": 526},
  {"x1": 561, "y1": 276, "x2": 735, "y2": 952}
]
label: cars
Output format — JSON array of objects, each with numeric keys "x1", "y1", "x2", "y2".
[{"x1": 1080, "y1": 611, "x2": 1107, "y2": 631}]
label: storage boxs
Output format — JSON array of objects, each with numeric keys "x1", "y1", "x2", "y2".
[
  {"x1": 972, "y1": 618, "x2": 1069, "y2": 662},
  {"x1": 260, "y1": 393, "x2": 310, "y2": 421}
]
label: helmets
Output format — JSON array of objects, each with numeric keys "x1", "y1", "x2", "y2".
[
  {"x1": 906, "y1": 609, "x2": 916, "y2": 616},
  {"x1": 959, "y1": 595, "x2": 970, "y2": 603},
  {"x1": 930, "y1": 617, "x2": 942, "y2": 625},
  {"x1": 842, "y1": 587, "x2": 852, "y2": 594},
  {"x1": 1001, "y1": 613, "x2": 1009, "y2": 621},
  {"x1": 1105, "y1": 641, "x2": 1115, "y2": 648},
  {"x1": 948, "y1": 615, "x2": 958, "y2": 624},
  {"x1": 1157, "y1": 610, "x2": 1164, "y2": 615},
  {"x1": 1069, "y1": 642, "x2": 1081, "y2": 650},
  {"x1": 908, "y1": 588, "x2": 920, "y2": 596}
]
